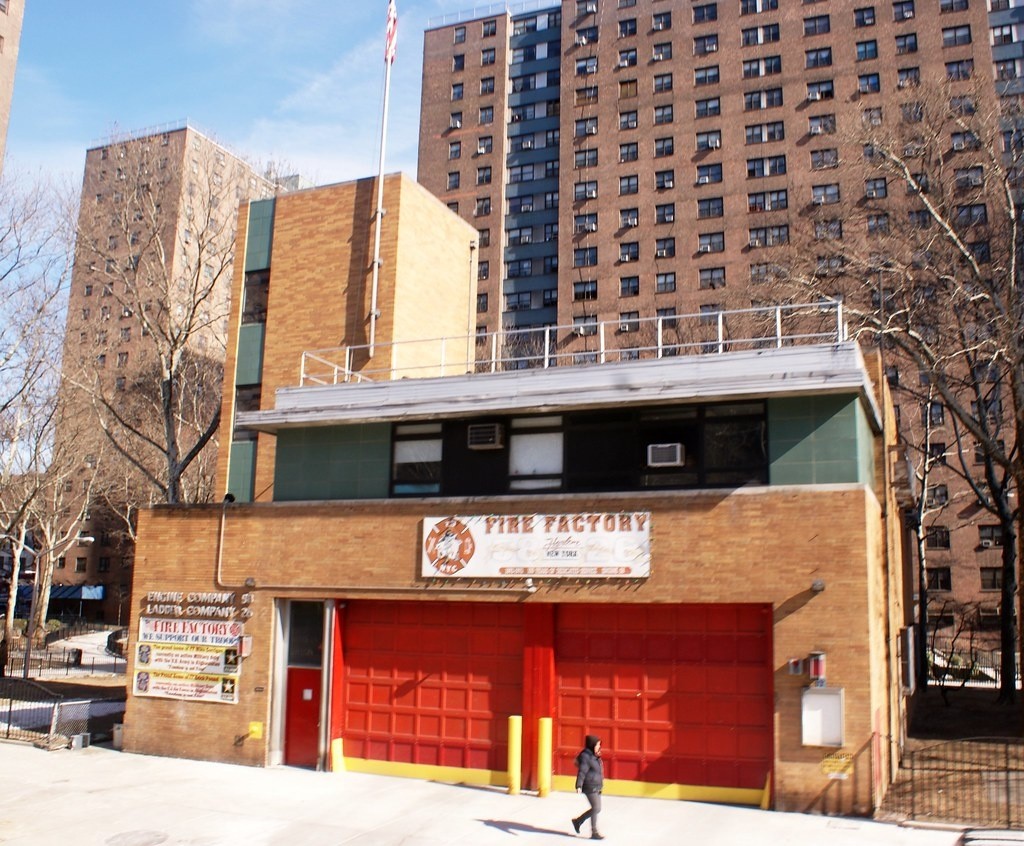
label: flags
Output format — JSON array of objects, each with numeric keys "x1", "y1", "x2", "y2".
[{"x1": 384, "y1": 0, "x2": 396, "y2": 65}]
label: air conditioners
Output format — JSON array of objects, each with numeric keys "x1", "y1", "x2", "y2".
[
  {"x1": 467, "y1": 423, "x2": 504, "y2": 450},
  {"x1": 448, "y1": 0, "x2": 987, "y2": 335},
  {"x1": 983, "y1": 539, "x2": 991, "y2": 547},
  {"x1": 648, "y1": 443, "x2": 685, "y2": 467},
  {"x1": 979, "y1": 497, "x2": 989, "y2": 504}
]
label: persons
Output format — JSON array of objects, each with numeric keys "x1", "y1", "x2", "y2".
[{"x1": 572, "y1": 734, "x2": 605, "y2": 841}]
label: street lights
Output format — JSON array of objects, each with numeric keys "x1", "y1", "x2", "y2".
[{"x1": 23, "y1": 537, "x2": 94, "y2": 679}]
